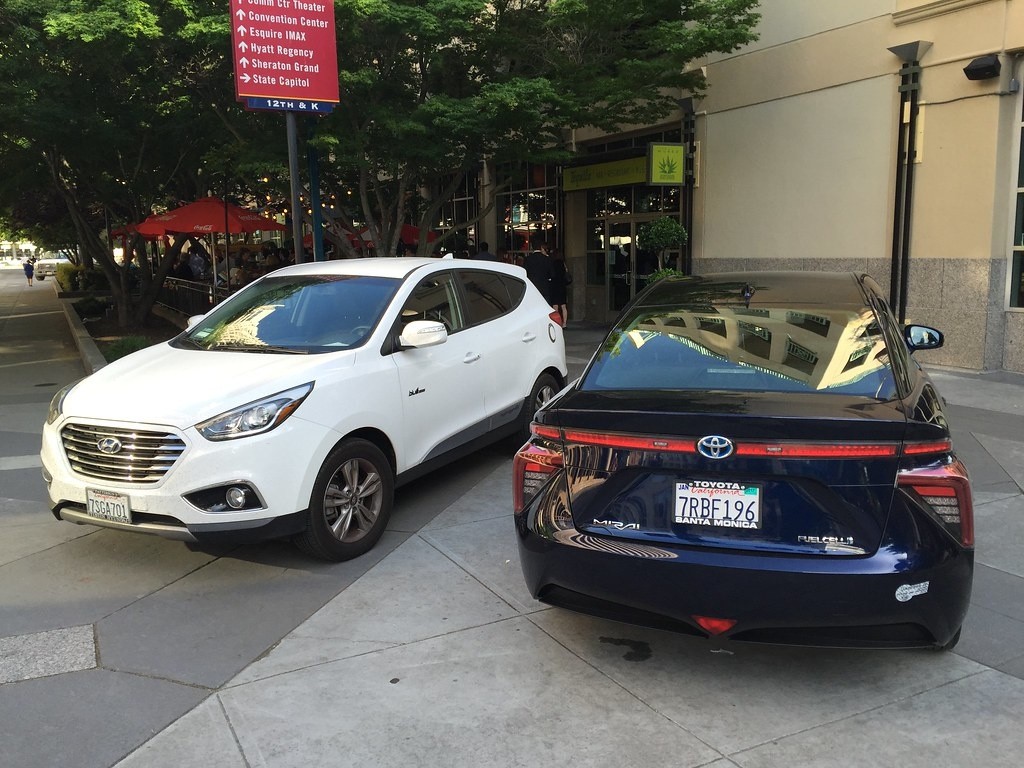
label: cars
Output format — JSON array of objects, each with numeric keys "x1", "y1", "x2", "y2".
[
  {"x1": 34, "y1": 249, "x2": 78, "y2": 281},
  {"x1": 511, "y1": 270, "x2": 976, "y2": 650},
  {"x1": 39, "y1": 256, "x2": 574, "y2": 560}
]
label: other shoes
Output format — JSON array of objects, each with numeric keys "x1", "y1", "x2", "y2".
[{"x1": 563, "y1": 327, "x2": 567, "y2": 330}]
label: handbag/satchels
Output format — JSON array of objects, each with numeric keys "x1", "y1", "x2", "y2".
[{"x1": 564, "y1": 262, "x2": 573, "y2": 287}]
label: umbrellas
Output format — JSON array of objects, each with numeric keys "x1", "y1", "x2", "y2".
[
  {"x1": 302, "y1": 226, "x2": 354, "y2": 247},
  {"x1": 108, "y1": 196, "x2": 290, "y2": 305},
  {"x1": 350, "y1": 222, "x2": 436, "y2": 248}
]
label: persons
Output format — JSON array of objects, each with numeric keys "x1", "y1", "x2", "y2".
[
  {"x1": 21, "y1": 254, "x2": 36, "y2": 286},
  {"x1": 177, "y1": 239, "x2": 573, "y2": 329}
]
